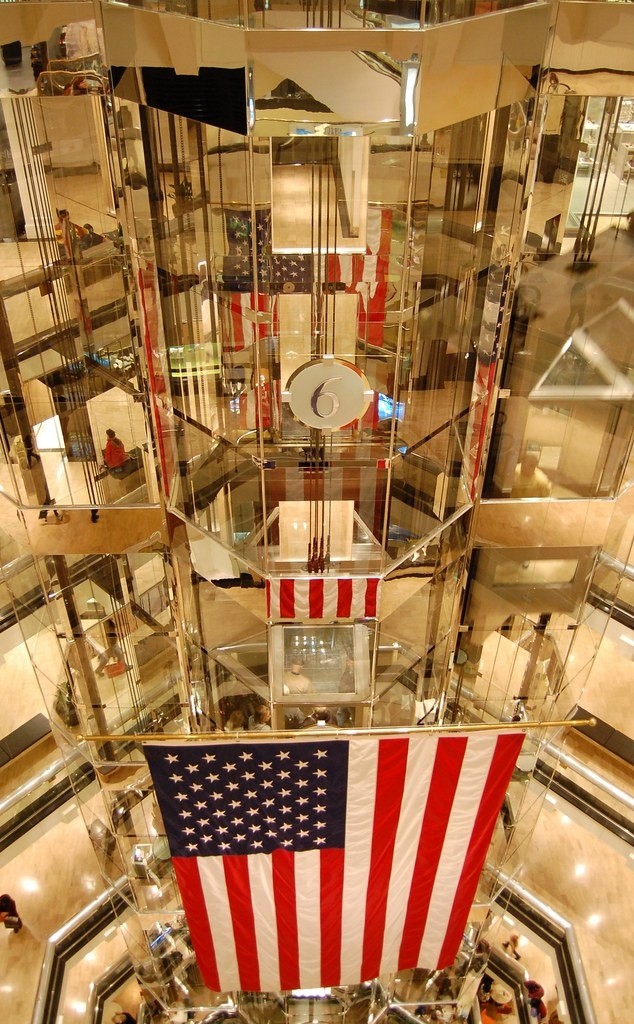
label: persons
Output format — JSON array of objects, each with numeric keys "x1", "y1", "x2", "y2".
[
  {"x1": 105, "y1": 429, "x2": 128, "y2": 467},
  {"x1": 21, "y1": 435, "x2": 40, "y2": 470},
  {"x1": 510, "y1": 451, "x2": 552, "y2": 567},
  {"x1": 54, "y1": 208, "x2": 105, "y2": 258},
  {"x1": 225, "y1": 650, "x2": 355, "y2": 732},
  {"x1": 94, "y1": 617, "x2": 133, "y2": 677},
  {"x1": 0, "y1": 894, "x2": 22, "y2": 933},
  {"x1": 502, "y1": 934, "x2": 521, "y2": 960},
  {"x1": 184, "y1": 998, "x2": 198, "y2": 1019},
  {"x1": 113, "y1": 1012, "x2": 137, "y2": 1024},
  {"x1": 40, "y1": 481, "x2": 63, "y2": 523},
  {"x1": 415, "y1": 939, "x2": 564, "y2": 1024},
  {"x1": 91, "y1": 510, "x2": 99, "y2": 524}
]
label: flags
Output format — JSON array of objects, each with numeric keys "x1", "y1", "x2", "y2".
[
  {"x1": 265, "y1": 577, "x2": 381, "y2": 618},
  {"x1": 221, "y1": 207, "x2": 392, "y2": 292},
  {"x1": 142, "y1": 727, "x2": 527, "y2": 993}
]
